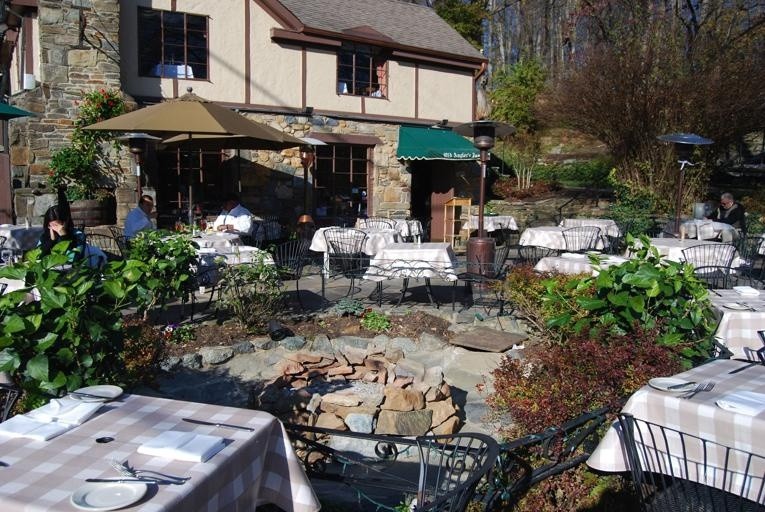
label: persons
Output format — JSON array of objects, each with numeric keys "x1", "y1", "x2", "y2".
[
  {"x1": 125, "y1": 193, "x2": 159, "y2": 249},
  {"x1": 704, "y1": 191, "x2": 746, "y2": 245},
  {"x1": 213, "y1": 197, "x2": 253, "y2": 246},
  {"x1": 34, "y1": 206, "x2": 86, "y2": 260}
]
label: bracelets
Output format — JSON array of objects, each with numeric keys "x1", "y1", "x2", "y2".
[{"x1": 226, "y1": 225, "x2": 229, "y2": 230}]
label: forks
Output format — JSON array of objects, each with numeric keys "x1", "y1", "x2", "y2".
[
  {"x1": 680, "y1": 382, "x2": 715, "y2": 400},
  {"x1": 110, "y1": 461, "x2": 186, "y2": 485}
]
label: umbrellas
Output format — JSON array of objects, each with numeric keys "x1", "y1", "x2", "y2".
[
  {"x1": 81, "y1": 87, "x2": 313, "y2": 226},
  {"x1": 160, "y1": 128, "x2": 310, "y2": 208},
  {"x1": 0, "y1": 101, "x2": 34, "y2": 120}
]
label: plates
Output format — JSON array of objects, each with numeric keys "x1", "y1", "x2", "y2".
[
  {"x1": 648, "y1": 376, "x2": 697, "y2": 392},
  {"x1": 69, "y1": 385, "x2": 123, "y2": 403},
  {"x1": 70, "y1": 476, "x2": 148, "y2": 512},
  {"x1": 723, "y1": 302, "x2": 752, "y2": 310}
]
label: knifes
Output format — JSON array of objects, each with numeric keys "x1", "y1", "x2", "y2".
[
  {"x1": 67, "y1": 392, "x2": 125, "y2": 402},
  {"x1": 728, "y1": 362, "x2": 756, "y2": 375},
  {"x1": 667, "y1": 382, "x2": 696, "y2": 390},
  {"x1": 85, "y1": 478, "x2": 157, "y2": 484},
  {"x1": 182, "y1": 418, "x2": 255, "y2": 432}
]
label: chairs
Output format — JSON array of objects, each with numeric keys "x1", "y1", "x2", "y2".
[
  {"x1": 518, "y1": 245, "x2": 560, "y2": 266},
  {"x1": 311, "y1": 213, "x2": 764, "y2": 249},
  {"x1": 323, "y1": 228, "x2": 366, "y2": 279},
  {"x1": 269, "y1": 239, "x2": 310, "y2": 315},
  {"x1": 693, "y1": 265, "x2": 752, "y2": 288},
  {"x1": 451, "y1": 236, "x2": 511, "y2": 310},
  {"x1": 681, "y1": 244, "x2": 738, "y2": 282},
  {"x1": 329, "y1": 239, "x2": 382, "y2": 306},
  {"x1": 613, "y1": 412, "x2": 764, "y2": 511},
  {"x1": 726, "y1": 237, "x2": 764, "y2": 286},
  {"x1": 306, "y1": 433, "x2": 499, "y2": 512}
]
